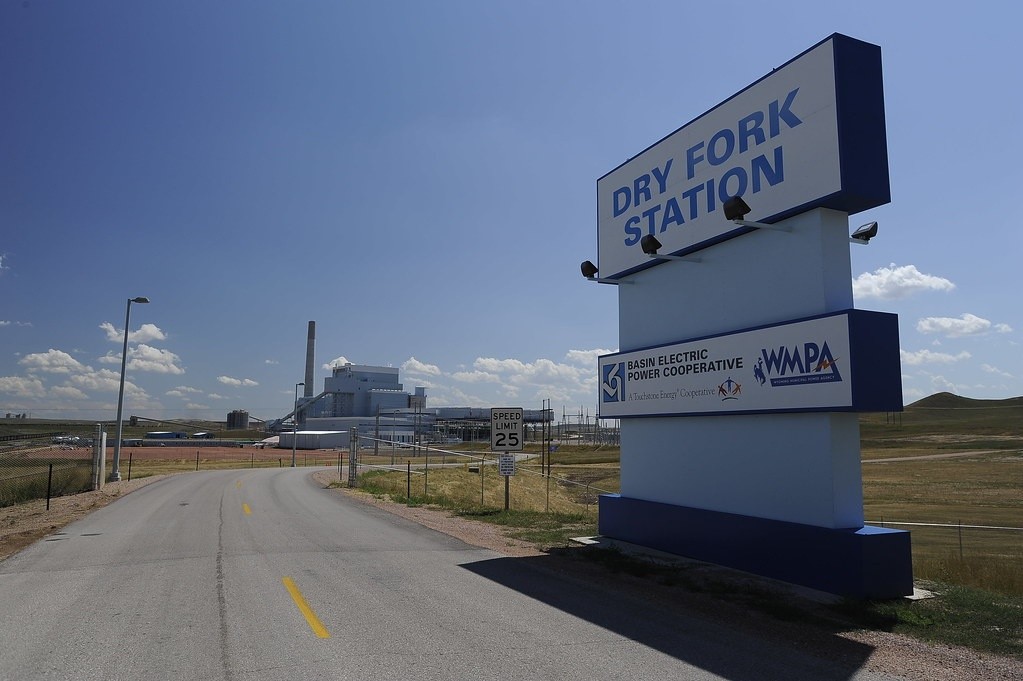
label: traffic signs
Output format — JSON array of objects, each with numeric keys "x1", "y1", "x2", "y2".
[{"x1": 491, "y1": 405, "x2": 525, "y2": 459}]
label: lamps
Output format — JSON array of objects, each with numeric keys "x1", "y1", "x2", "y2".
[
  {"x1": 581, "y1": 261, "x2": 622, "y2": 284},
  {"x1": 849, "y1": 221, "x2": 878, "y2": 245},
  {"x1": 641, "y1": 234, "x2": 702, "y2": 263},
  {"x1": 723, "y1": 197, "x2": 794, "y2": 233}
]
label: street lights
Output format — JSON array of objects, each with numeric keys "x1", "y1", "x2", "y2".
[
  {"x1": 292, "y1": 382, "x2": 304, "y2": 467},
  {"x1": 105, "y1": 295, "x2": 150, "y2": 481}
]
